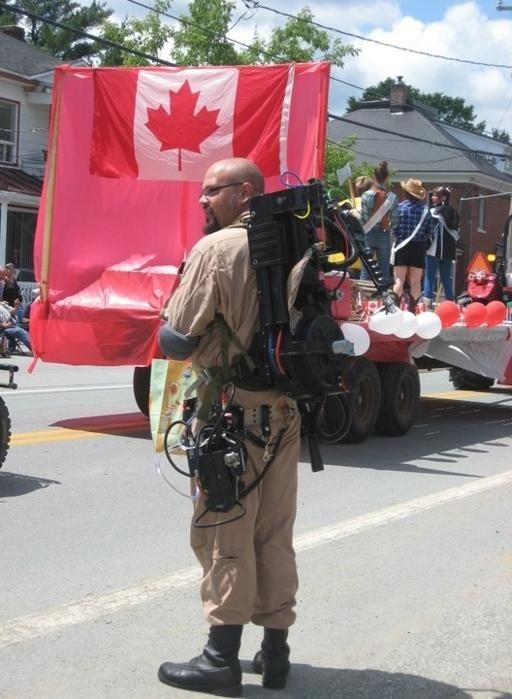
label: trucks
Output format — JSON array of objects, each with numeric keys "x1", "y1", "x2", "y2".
[{"x1": 133, "y1": 196, "x2": 512, "y2": 445}]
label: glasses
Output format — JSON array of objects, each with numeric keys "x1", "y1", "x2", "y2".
[{"x1": 204, "y1": 183, "x2": 243, "y2": 197}]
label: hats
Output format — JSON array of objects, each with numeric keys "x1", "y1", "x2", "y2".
[{"x1": 399, "y1": 177, "x2": 427, "y2": 200}]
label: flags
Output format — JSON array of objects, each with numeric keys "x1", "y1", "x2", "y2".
[{"x1": 27, "y1": 61, "x2": 330, "y2": 369}]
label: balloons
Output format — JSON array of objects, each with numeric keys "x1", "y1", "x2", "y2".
[
  {"x1": 434, "y1": 298, "x2": 461, "y2": 329},
  {"x1": 365, "y1": 310, "x2": 400, "y2": 340},
  {"x1": 484, "y1": 296, "x2": 507, "y2": 330},
  {"x1": 340, "y1": 322, "x2": 371, "y2": 356},
  {"x1": 462, "y1": 300, "x2": 487, "y2": 330},
  {"x1": 415, "y1": 311, "x2": 442, "y2": 342},
  {"x1": 389, "y1": 310, "x2": 416, "y2": 339}
]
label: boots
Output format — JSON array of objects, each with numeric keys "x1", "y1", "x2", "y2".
[
  {"x1": 158, "y1": 624, "x2": 242, "y2": 696},
  {"x1": 253, "y1": 628, "x2": 290, "y2": 689}
]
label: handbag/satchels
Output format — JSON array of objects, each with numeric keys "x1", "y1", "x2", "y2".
[{"x1": 149, "y1": 358, "x2": 196, "y2": 455}]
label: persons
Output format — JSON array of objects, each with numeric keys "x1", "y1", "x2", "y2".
[
  {"x1": 0, "y1": 298, "x2": 35, "y2": 352},
  {"x1": 0, "y1": 266, "x2": 7, "y2": 302},
  {"x1": 155, "y1": 157, "x2": 297, "y2": 695},
  {"x1": 358, "y1": 160, "x2": 401, "y2": 283},
  {"x1": 422, "y1": 184, "x2": 462, "y2": 303},
  {"x1": 1, "y1": 262, "x2": 25, "y2": 305},
  {"x1": 387, "y1": 178, "x2": 432, "y2": 300},
  {"x1": 329, "y1": 174, "x2": 374, "y2": 280}
]
